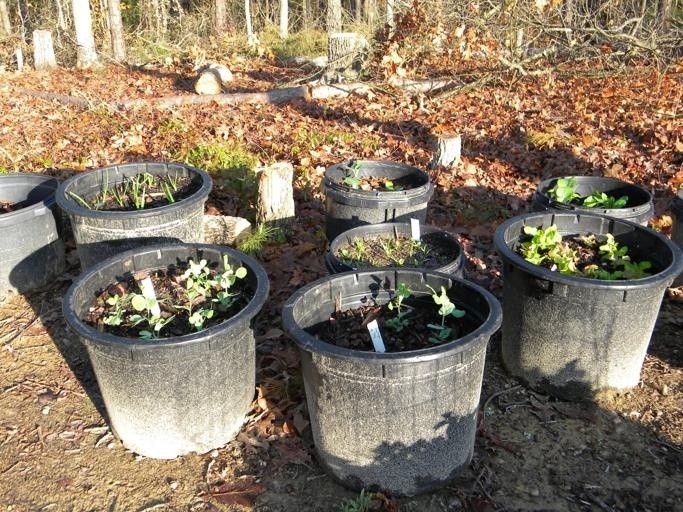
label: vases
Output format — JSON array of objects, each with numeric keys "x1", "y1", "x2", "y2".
[{"x1": 1, "y1": 175, "x2": 60, "y2": 295}]
